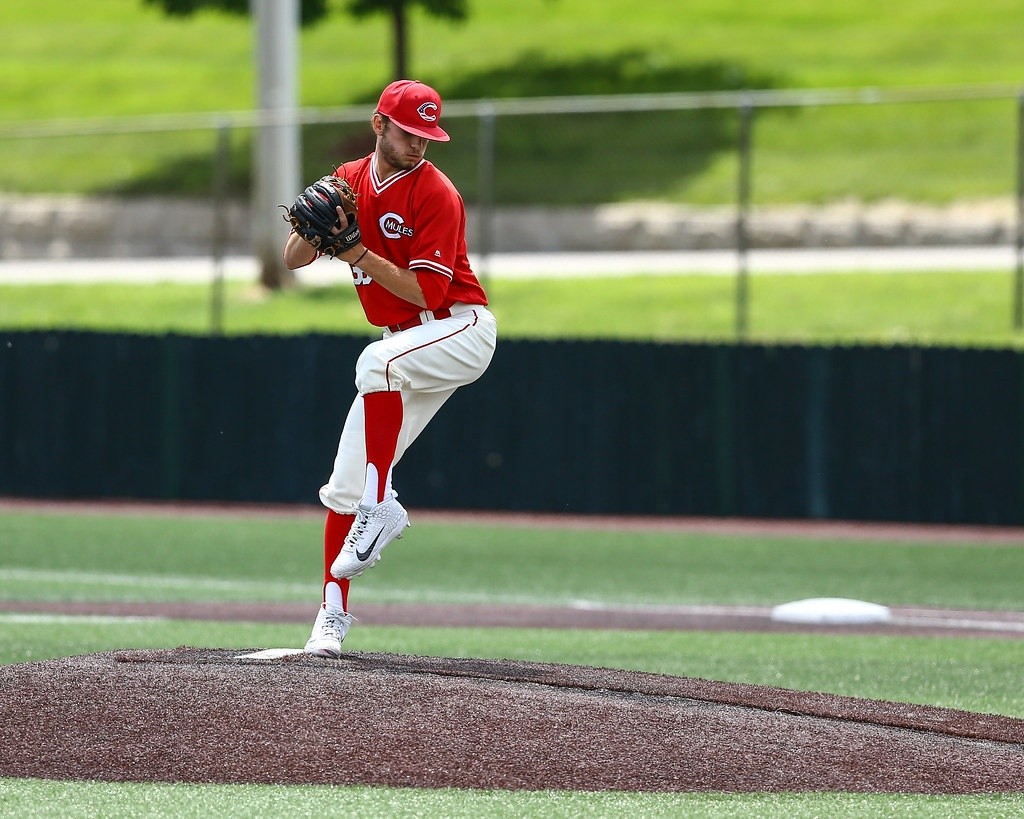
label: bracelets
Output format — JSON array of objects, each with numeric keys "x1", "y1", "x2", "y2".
[{"x1": 350, "y1": 247, "x2": 369, "y2": 267}]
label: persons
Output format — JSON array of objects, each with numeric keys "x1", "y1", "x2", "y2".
[{"x1": 283, "y1": 80, "x2": 498, "y2": 656}]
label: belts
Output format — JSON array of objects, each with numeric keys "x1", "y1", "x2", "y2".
[{"x1": 388, "y1": 306, "x2": 452, "y2": 333}]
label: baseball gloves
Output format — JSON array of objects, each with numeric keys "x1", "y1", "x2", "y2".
[{"x1": 287, "y1": 175, "x2": 362, "y2": 257}]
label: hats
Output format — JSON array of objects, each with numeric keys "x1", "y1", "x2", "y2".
[{"x1": 375, "y1": 80, "x2": 451, "y2": 142}]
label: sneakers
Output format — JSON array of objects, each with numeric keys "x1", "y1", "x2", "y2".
[
  {"x1": 330, "y1": 490, "x2": 410, "y2": 581},
  {"x1": 303, "y1": 602, "x2": 359, "y2": 658}
]
